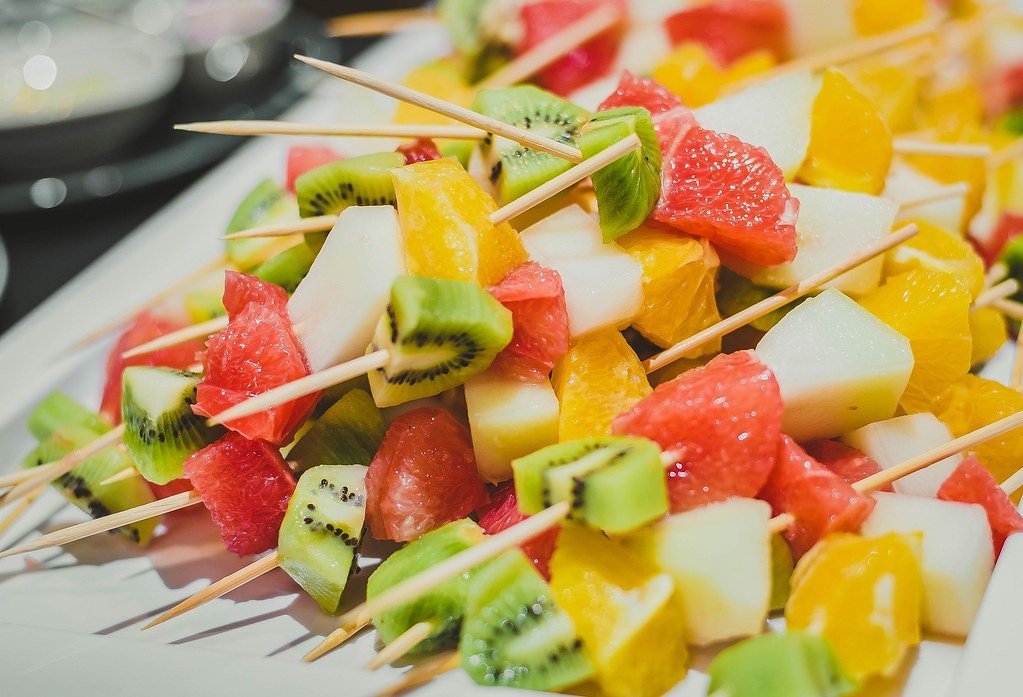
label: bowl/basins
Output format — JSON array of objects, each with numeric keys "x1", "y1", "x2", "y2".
[
  {"x1": 1, "y1": 39, "x2": 182, "y2": 176},
  {"x1": 169, "y1": 1, "x2": 293, "y2": 95}
]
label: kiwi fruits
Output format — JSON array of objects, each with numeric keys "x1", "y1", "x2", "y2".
[{"x1": 20, "y1": 0, "x2": 855, "y2": 697}]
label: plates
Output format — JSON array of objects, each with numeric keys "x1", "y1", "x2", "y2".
[
  {"x1": 1, "y1": 0, "x2": 1023, "y2": 697},
  {"x1": 1, "y1": 0, "x2": 340, "y2": 211}
]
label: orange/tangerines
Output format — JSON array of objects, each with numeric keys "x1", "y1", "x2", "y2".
[{"x1": 386, "y1": 0, "x2": 1023, "y2": 690}]
